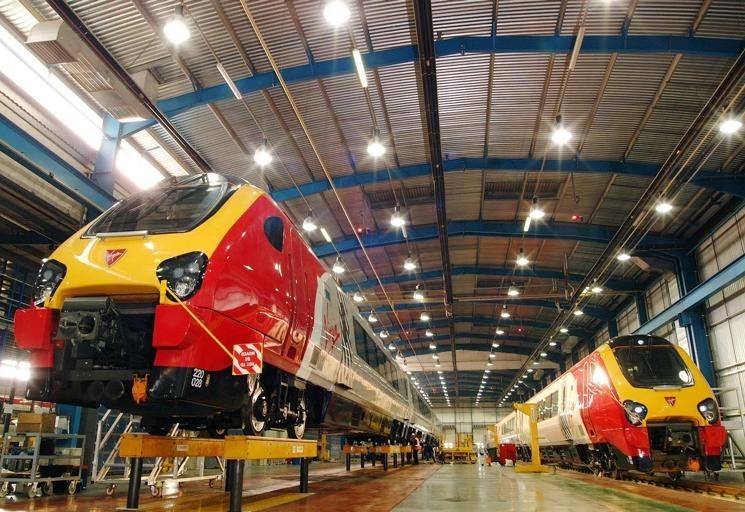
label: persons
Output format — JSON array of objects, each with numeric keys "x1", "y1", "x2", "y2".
[{"x1": 411, "y1": 432, "x2": 436, "y2": 465}]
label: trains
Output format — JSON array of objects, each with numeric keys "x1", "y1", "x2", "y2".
[
  {"x1": 484, "y1": 335, "x2": 727, "y2": 480},
  {"x1": 12, "y1": 173, "x2": 445, "y2": 449}
]
